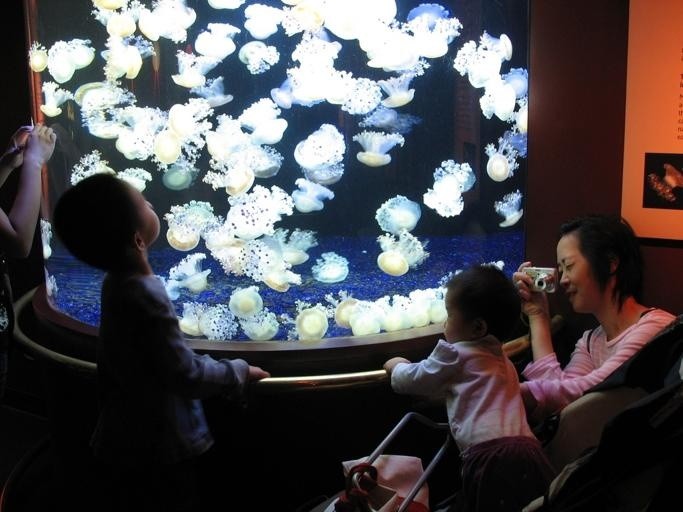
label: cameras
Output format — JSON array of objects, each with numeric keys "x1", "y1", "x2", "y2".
[{"x1": 522, "y1": 268, "x2": 558, "y2": 294}]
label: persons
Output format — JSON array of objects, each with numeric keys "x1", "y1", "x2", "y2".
[
  {"x1": 510, "y1": 210, "x2": 683, "y2": 434},
  {"x1": 1, "y1": 120, "x2": 60, "y2": 424},
  {"x1": 382, "y1": 261, "x2": 562, "y2": 511},
  {"x1": 49, "y1": 171, "x2": 274, "y2": 512}
]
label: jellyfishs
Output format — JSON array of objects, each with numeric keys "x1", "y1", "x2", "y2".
[{"x1": 26, "y1": 0, "x2": 528, "y2": 345}]
